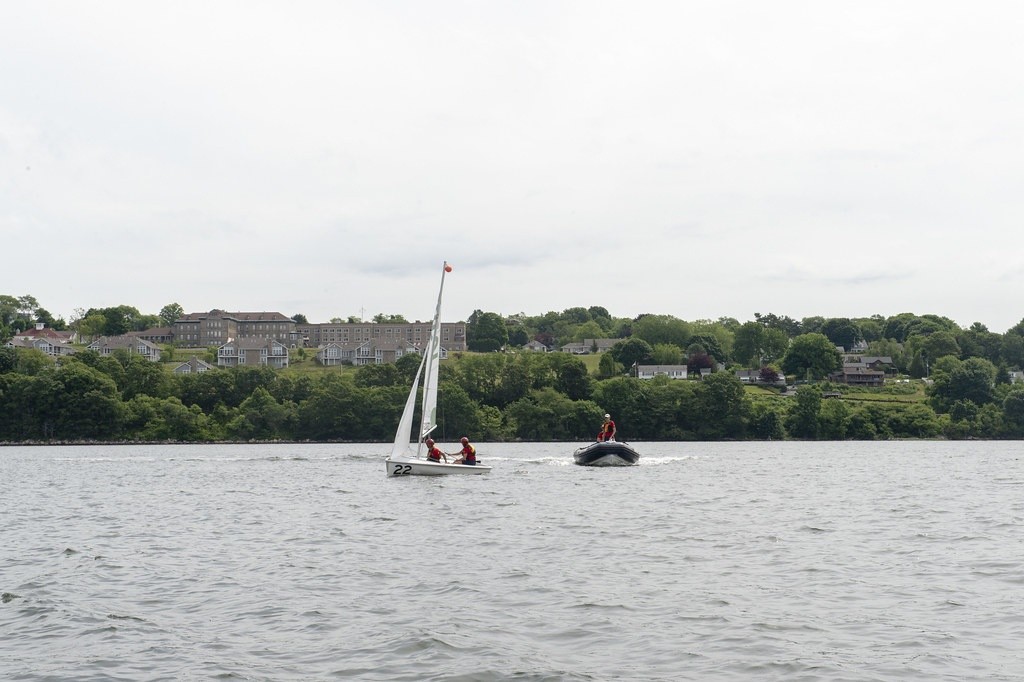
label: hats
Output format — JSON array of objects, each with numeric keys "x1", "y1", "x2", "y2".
[
  {"x1": 601, "y1": 424, "x2": 604, "y2": 428},
  {"x1": 605, "y1": 414, "x2": 610, "y2": 418}
]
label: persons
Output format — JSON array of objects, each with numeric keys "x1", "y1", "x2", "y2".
[
  {"x1": 448, "y1": 437, "x2": 476, "y2": 465},
  {"x1": 597, "y1": 413, "x2": 616, "y2": 443},
  {"x1": 426, "y1": 439, "x2": 446, "y2": 463}
]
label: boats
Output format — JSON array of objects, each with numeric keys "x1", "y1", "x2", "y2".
[{"x1": 574, "y1": 441, "x2": 640, "y2": 466}]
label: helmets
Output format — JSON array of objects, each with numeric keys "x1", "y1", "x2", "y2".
[
  {"x1": 426, "y1": 439, "x2": 434, "y2": 448},
  {"x1": 461, "y1": 437, "x2": 468, "y2": 447}
]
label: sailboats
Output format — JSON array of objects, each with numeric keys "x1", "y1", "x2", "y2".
[{"x1": 385, "y1": 261, "x2": 494, "y2": 476}]
